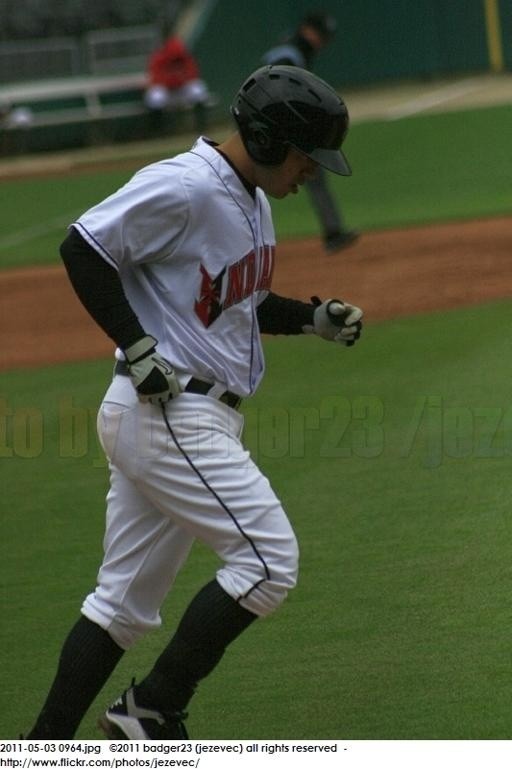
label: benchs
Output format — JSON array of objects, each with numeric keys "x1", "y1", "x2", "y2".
[{"x1": 0, "y1": 59, "x2": 219, "y2": 151}]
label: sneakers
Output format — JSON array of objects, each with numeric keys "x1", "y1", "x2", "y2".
[
  {"x1": 97, "y1": 676, "x2": 190, "y2": 739},
  {"x1": 325, "y1": 227, "x2": 360, "y2": 253}
]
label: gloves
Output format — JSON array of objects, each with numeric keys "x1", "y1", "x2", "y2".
[
  {"x1": 310, "y1": 295, "x2": 364, "y2": 346},
  {"x1": 125, "y1": 334, "x2": 184, "y2": 406}
]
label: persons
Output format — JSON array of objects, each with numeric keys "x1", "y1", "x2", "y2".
[
  {"x1": 142, "y1": 37, "x2": 212, "y2": 137},
  {"x1": 18, "y1": 63, "x2": 363, "y2": 739},
  {"x1": 257, "y1": 13, "x2": 363, "y2": 256}
]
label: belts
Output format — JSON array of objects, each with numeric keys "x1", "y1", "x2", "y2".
[{"x1": 116, "y1": 360, "x2": 242, "y2": 411}]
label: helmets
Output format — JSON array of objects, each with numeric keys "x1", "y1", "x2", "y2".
[{"x1": 231, "y1": 64, "x2": 353, "y2": 177}]
